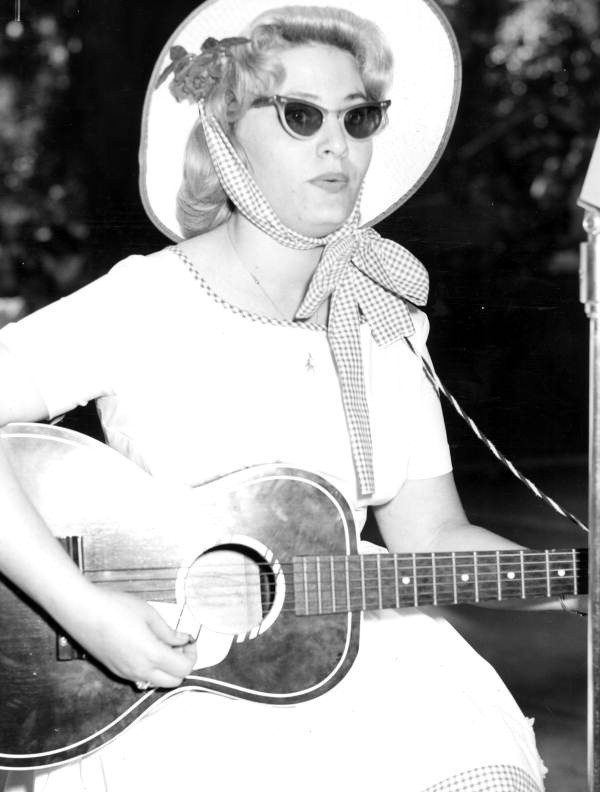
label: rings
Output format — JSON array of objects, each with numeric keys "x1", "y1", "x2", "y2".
[{"x1": 135, "y1": 680, "x2": 151, "y2": 690}]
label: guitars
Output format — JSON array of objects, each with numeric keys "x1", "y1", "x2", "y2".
[{"x1": 0, "y1": 420, "x2": 589, "y2": 774}]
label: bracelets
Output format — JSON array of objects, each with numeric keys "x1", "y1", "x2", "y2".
[{"x1": 559, "y1": 596, "x2": 588, "y2": 617}]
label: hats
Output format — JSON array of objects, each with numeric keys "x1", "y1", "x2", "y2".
[{"x1": 138, "y1": 1, "x2": 462, "y2": 244}]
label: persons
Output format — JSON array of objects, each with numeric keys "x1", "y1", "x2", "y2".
[{"x1": 0, "y1": 0, "x2": 589, "y2": 792}]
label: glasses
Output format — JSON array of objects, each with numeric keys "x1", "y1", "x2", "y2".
[{"x1": 251, "y1": 94, "x2": 391, "y2": 141}]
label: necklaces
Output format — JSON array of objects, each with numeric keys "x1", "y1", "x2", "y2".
[{"x1": 226, "y1": 222, "x2": 321, "y2": 324}]
